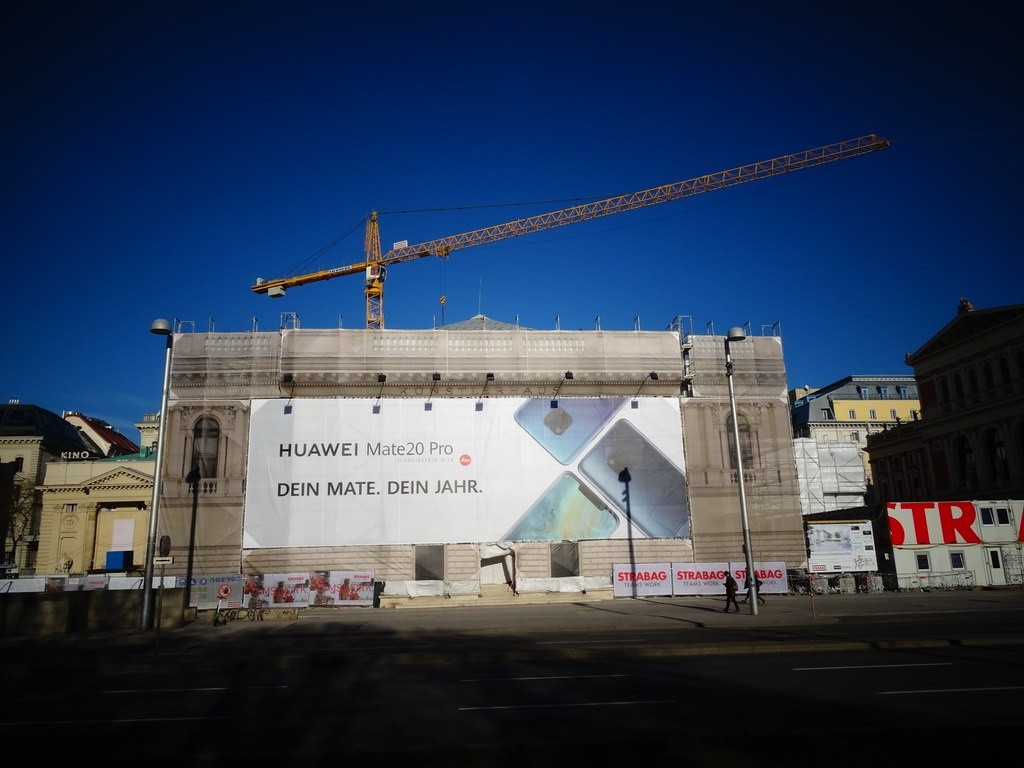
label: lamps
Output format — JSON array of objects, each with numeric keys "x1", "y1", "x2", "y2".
[
  {"x1": 378, "y1": 375, "x2": 386, "y2": 389},
  {"x1": 283, "y1": 374, "x2": 296, "y2": 390},
  {"x1": 642, "y1": 371, "x2": 659, "y2": 384},
  {"x1": 561, "y1": 371, "x2": 573, "y2": 382},
  {"x1": 432, "y1": 373, "x2": 440, "y2": 388},
  {"x1": 484, "y1": 373, "x2": 495, "y2": 387}
]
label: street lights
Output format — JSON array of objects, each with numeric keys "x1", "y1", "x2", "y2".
[
  {"x1": 140, "y1": 317, "x2": 176, "y2": 633},
  {"x1": 724, "y1": 327, "x2": 757, "y2": 615}
]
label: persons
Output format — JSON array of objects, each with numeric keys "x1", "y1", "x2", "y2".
[
  {"x1": 742, "y1": 573, "x2": 765, "y2": 605},
  {"x1": 722, "y1": 571, "x2": 740, "y2": 613},
  {"x1": 245, "y1": 575, "x2": 360, "y2": 608}
]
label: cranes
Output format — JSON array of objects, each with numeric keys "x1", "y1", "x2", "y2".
[{"x1": 250, "y1": 133, "x2": 894, "y2": 330}]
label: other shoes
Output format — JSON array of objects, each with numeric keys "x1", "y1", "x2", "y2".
[
  {"x1": 742, "y1": 600, "x2": 746, "y2": 603},
  {"x1": 723, "y1": 608, "x2": 728, "y2": 613},
  {"x1": 735, "y1": 609, "x2": 739, "y2": 612},
  {"x1": 763, "y1": 599, "x2": 765, "y2": 604}
]
